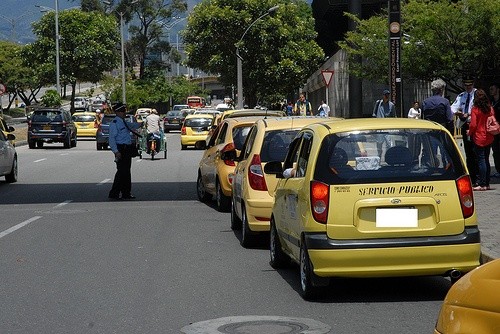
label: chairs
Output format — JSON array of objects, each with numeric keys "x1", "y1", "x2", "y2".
[
  {"x1": 379, "y1": 146, "x2": 412, "y2": 170},
  {"x1": 260, "y1": 135, "x2": 286, "y2": 162},
  {"x1": 330, "y1": 148, "x2": 353, "y2": 171}
]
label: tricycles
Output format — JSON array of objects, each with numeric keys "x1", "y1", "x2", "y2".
[{"x1": 140, "y1": 127, "x2": 168, "y2": 161}]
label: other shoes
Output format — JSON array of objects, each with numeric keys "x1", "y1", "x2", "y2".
[
  {"x1": 473, "y1": 185, "x2": 490, "y2": 191},
  {"x1": 108, "y1": 192, "x2": 137, "y2": 200}
]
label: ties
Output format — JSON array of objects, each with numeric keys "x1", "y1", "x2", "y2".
[{"x1": 464, "y1": 94, "x2": 470, "y2": 113}]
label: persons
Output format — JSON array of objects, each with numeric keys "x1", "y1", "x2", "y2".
[
  {"x1": 451, "y1": 76, "x2": 500, "y2": 191},
  {"x1": 408, "y1": 102, "x2": 421, "y2": 119},
  {"x1": 294, "y1": 93, "x2": 313, "y2": 116},
  {"x1": 95, "y1": 101, "x2": 108, "y2": 125},
  {"x1": 272, "y1": 102, "x2": 281, "y2": 110},
  {"x1": 110, "y1": 102, "x2": 144, "y2": 198},
  {"x1": 372, "y1": 90, "x2": 397, "y2": 161},
  {"x1": 421, "y1": 79, "x2": 455, "y2": 168},
  {"x1": 283, "y1": 168, "x2": 295, "y2": 178},
  {"x1": 318, "y1": 99, "x2": 330, "y2": 117},
  {"x1": 216, "y1": 98, "x2": 234, "y2": 110},
  {"x1": 127, "y1": 104, "x2": 138, "y2": 115},
  {"x1": 145, "y1": 109, "x2": 163, "y2": 154},
  {"x1": 282, "y1": 100, "x2": 294, "y2": 116}
]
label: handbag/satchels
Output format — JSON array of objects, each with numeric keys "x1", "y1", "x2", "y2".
[{"x1": 486, "y1": 115, "x2": 500, "y2": 135}]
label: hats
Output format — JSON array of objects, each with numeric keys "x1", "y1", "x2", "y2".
[
  {"x1": 113, "y1": 103, "x2": 127, "y2": 112},
  {"x1": 383, "y1": 90, "x2": 391, "y2": 95},
  {"x1": 461, "y1": 79, "x2": 474, "y2": 86}
]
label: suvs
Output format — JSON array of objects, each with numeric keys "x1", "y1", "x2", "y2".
[{"x1": 27, "y1": 108, "x2": 78, "y2": 149}]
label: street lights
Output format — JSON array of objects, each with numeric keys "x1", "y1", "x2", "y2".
[
  {"x1": 34, "y1": 0, "x2": 81, "y2": 95},
  {"x1": 0, "y1": 13, "x2": 28, "y2": 41},
  {"x1": 237, "y1": 5, "x2": 280, "y2": 109},
  {"x1": 141, "y1": 17, "x2": 187, "y2": 82},
  {"x1": 102, "y1": 0, "x2": 139, "y2": 105}
]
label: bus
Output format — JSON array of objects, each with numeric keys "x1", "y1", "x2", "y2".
[{"x1": 186, "y1": 96, "x2": 206, "y2": 107}]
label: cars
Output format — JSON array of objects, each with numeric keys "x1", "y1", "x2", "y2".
[
  {"x1": 231, "y1": 115, "x2": 367, "y2": 247},
  {"x1": 0, "y1": 117, "x2": 18, "y2": 182},
  {"x1": 164, "y1": 105, "x2": 287, "y2": 150},
  {"x1": 95, "y1": 114, "x2": 137, "y2": 150},
  {"x1": 263, "y1": 113, "x2": 483, "y2": 300},
  {"x1": 72, "y1": 97, "x2": 108, "y2": 137},
  {"x1": 196, "y1": 115, "x2": 285, "y2": 212}
]
